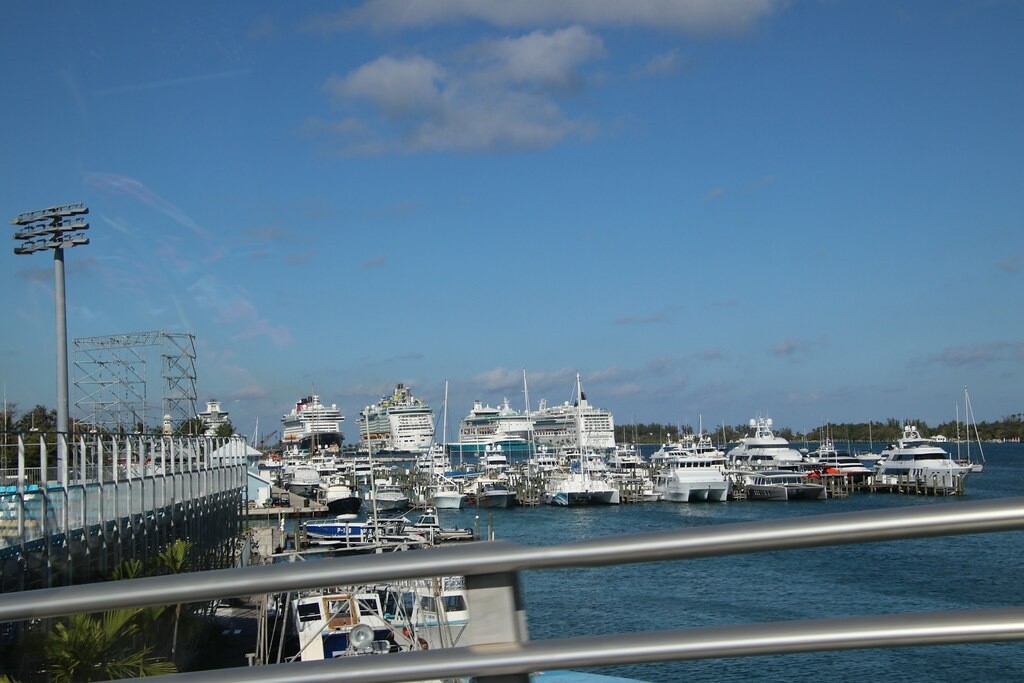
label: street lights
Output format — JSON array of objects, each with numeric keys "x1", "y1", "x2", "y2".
[{"x1": 12, "y1": 203, "x2": 91, "y2": 485}]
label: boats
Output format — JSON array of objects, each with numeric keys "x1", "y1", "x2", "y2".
[{"x1": 242, "y1": 365, "x2": 985, "y2": 683}]
